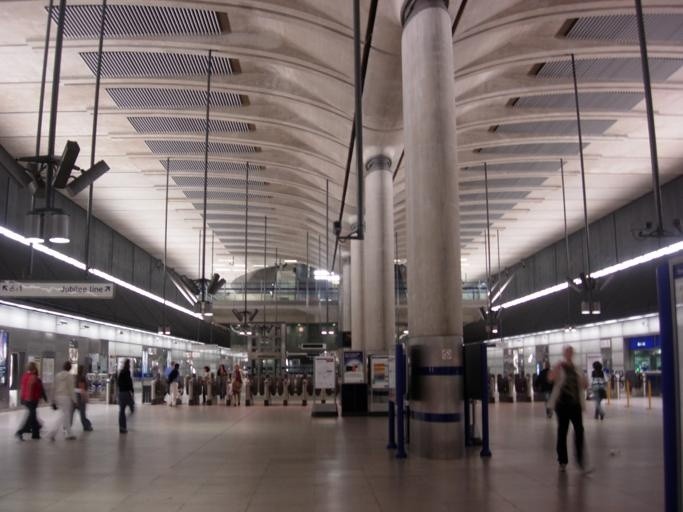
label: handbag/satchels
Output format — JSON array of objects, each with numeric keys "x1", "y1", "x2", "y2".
[{"x1": 597, "y1": 386, "x2": 608, "y2": 398}]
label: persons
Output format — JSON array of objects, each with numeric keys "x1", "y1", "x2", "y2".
[
  {"x1": 588, "y1": 360, "x2": 608, "y2": 420},
  {"x1": 14, "y1": 358, "x2": 134, "y2": 441},
  {"x1": 548, "y1": 346, "x2": 595, "y2": 475},
  {"x1": 163, "y1": 359, "x2": 242, "y2": 407},
  {"x1": 533, "y1": 360, "x2": 554, "y2": 417}
]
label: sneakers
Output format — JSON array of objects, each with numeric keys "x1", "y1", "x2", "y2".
[{"x1": 15, "y1": 426, "x2": 129, "y2": 443}]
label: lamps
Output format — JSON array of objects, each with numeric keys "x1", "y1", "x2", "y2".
[
  {"x1": 568, "y1": 53, "x2": 612, "y2": 314},
  {"x1": 479, "y1": 164, "x2": 504, "y2": 334},
  {"x1": 1, "y1": 1, "x2": 110, "y2": 244},
  {"x1": 157, "y1": 49, "x2": 339, "y2": 335}
]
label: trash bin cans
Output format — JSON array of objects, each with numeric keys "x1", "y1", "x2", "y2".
[{"x1": 143, "y1": 385, "x2": 151, "y2": 403}]
